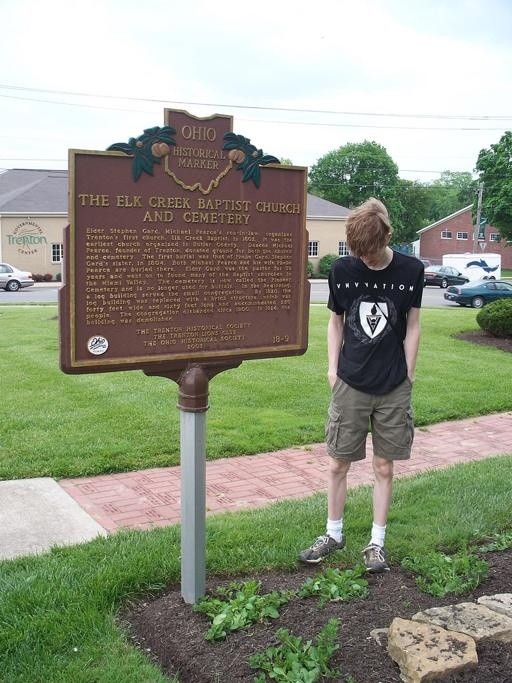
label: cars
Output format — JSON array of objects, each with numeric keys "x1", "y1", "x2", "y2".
[
  {"x1": 444, "y1": 279, "x2": 512, "y2": 308},
  {"x1": 0, "y1": 263, "x2": 34, "y2": 291},
  {"x1": 423, "y1": 265, "x2": 468, "y2": 288}
]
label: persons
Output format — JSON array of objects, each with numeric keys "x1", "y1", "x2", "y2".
[{"x1": 297, "y1": 197, "x2": 425, "y2": 573}]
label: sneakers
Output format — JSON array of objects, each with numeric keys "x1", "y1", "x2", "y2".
[
  {"x1": 299, "y1": 534, "x2": 347, "y2": 563},
  {"x1": 362, "y1": 543, "x2": 390, "y2": 573}
]
